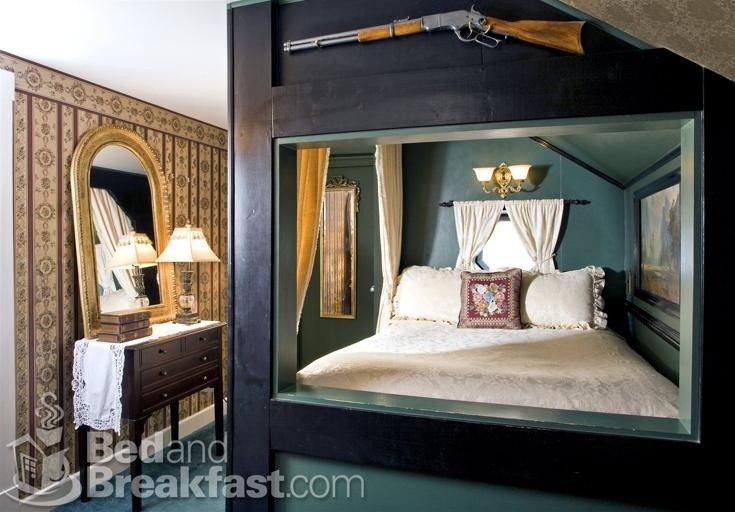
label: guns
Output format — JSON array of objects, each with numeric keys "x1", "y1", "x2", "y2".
[{"x1": 282, "y1": 5, "x2": 587, "y2": 57}]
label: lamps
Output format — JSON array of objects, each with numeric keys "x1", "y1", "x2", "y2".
[
  {"x1": 470, "y1": 160, "x2": 534, "y2": 200},
  {"x1": 105, "y1": 225, "x2": 159, "y2": 309},
  {"x1": 151, "y1": 219, "x2": 222, "y2": 327}
]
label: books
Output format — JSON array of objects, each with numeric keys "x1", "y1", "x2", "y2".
[{"x1": 97, "y1": 309, "x2": 152, "y2": 343}]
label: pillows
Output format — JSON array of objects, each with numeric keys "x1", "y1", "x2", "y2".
[{"x1": 387, "y1": 260, "x2": 610, "y2": 332}]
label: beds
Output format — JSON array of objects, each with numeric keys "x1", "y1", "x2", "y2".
[{"x1": 215, "y1": 0, "x2": 733, "y2": 512}]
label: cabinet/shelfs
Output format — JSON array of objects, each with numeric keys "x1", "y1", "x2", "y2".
[{"x1": 71, "y1": 316, "x2": 228, "y2": 511}]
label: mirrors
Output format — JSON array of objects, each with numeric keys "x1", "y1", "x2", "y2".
[
  {"x1": 317, "y1": 174, "x2": 361, "y2": 320},
  {"x1": 67, "y1": 122, "x2": 180, "y2": 339}
]
